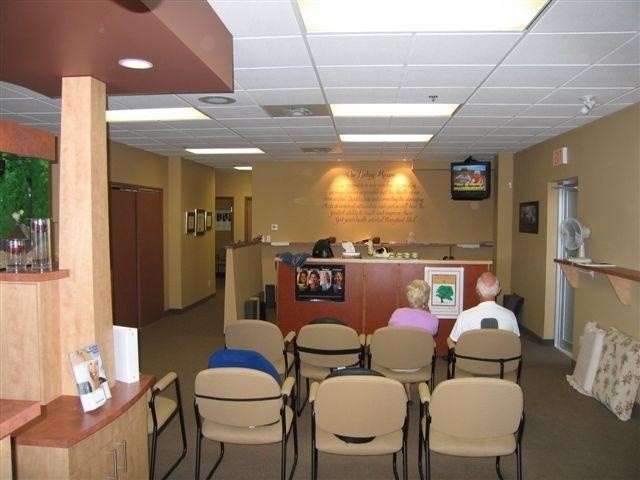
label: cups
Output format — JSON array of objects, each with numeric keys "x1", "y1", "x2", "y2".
[
  {"x1": 407, "y1": 231, "x2": 417, "y2": 244},
  {"x1": 388, "y1": 251, "x2": 420, "y2": 259},
  {"x1": 28, "y1": 216, "x2": 52, "y2": 271},
  {"x1": 4, "y1": 238, "x2": 26, "y2": 272}
]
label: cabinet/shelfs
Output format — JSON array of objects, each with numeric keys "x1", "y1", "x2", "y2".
[{"x1": 72, "y1": 394, "x2": 149, "y2": 480}]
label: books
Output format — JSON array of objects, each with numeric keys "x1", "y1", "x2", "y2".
[
  {"x1": 577, "y1": 261, "x2": 616, "y2": 267},
  {"x1": 68, "y1": 343, "x2": 112, "y2": 414}
]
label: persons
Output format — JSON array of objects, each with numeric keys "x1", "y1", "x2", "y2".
[
  {"x1": 456, "y1": 168, "x2": 472, "y2": 183},
  {"x1": 388, "y1": 278, "x2": 440, "y2": 405},
  {"x1": 297, "y1": 270, "x2": 309, "y2": 291},
  {"x1": 308, "y1": 270, "x2": 320, "y2": 291},
  {"x1": 321, "y1": 272, "x2": 332, "y2": 291},
  {"x1": 472, "y1": 170, "x2": 484, "y2": 184},
  {"x1": 75, "y1": 359, "x2": 107, "y2": 395},
  {"x1": 448, "y1": 269, "x2": 522, "y2": 345},
  {"x1": 332, "y1": 271, "x2": 343, "y2": 293}
]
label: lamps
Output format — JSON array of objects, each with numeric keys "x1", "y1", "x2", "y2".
[{"x1": 579, "y1": 99, "x2": 596, "y2": 115}]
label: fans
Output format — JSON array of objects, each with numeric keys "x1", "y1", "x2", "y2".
[{"x1": 558, "y1": 218, "x2": 593, "y2": 265}]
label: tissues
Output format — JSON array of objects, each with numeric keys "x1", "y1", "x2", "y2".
[{"x1": 340, "y1": 240, "x2": 362, "y2": 259}]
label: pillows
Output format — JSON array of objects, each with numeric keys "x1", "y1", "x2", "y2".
[{"x1": 564, "y1": 320, "x2": 639, "y2": 423}]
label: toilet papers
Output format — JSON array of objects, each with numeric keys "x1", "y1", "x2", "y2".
[
  {"x1": 270, "y1": 241, "x2": 290, "y2": 246},
  {"x1": 457, "y1": 242, "x2": 481, "y2": 249}
]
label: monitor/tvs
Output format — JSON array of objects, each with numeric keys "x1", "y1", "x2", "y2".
[{"x1": 450, "y1": 161, "x2": 491, "y2": 199}]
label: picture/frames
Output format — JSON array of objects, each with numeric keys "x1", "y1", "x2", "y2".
[
  {"x1": 423, "y1": 266, "x2": 465, "y2": 319},
  {"x1": 185, "y1": 209, "x2": 213, "y2": 238}
]
